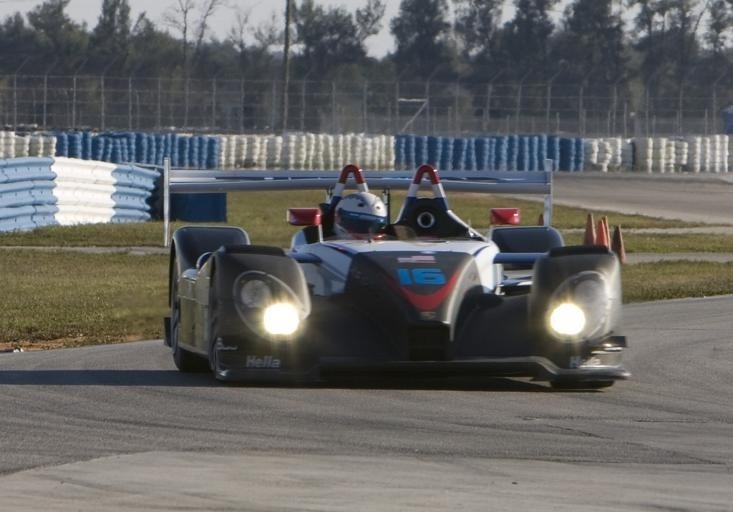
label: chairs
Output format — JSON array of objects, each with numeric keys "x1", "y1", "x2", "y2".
[{"x1": 405, "y1": 198, "x2": 448, "y2": 238}]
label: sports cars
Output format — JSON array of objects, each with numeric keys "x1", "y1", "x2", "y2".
[{"x1": 162, "y1": 162, "x2": 630, "y2": 394}]
